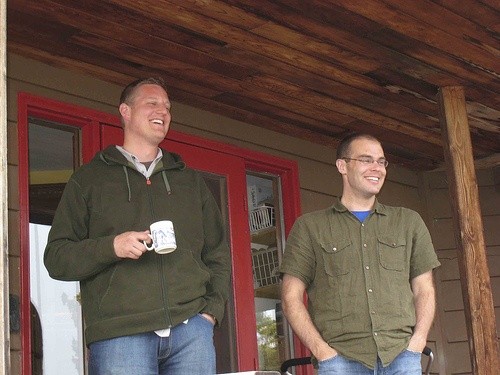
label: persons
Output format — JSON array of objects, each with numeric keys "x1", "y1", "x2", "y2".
[
  {"x1": 43, "y1": 75, "x2": 233, "y2": 375},
  {"x1": 278, "y1": 131, "x2": 442, "y2": 374}
]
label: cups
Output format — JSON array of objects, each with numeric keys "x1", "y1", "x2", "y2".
[{"x1": 143, "y1": 220, "x2": 177, "y2": 254}]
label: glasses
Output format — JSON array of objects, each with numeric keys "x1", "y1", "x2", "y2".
[{"x1": 342, "y1": 158, "x2": 388, "y2": 168}]
